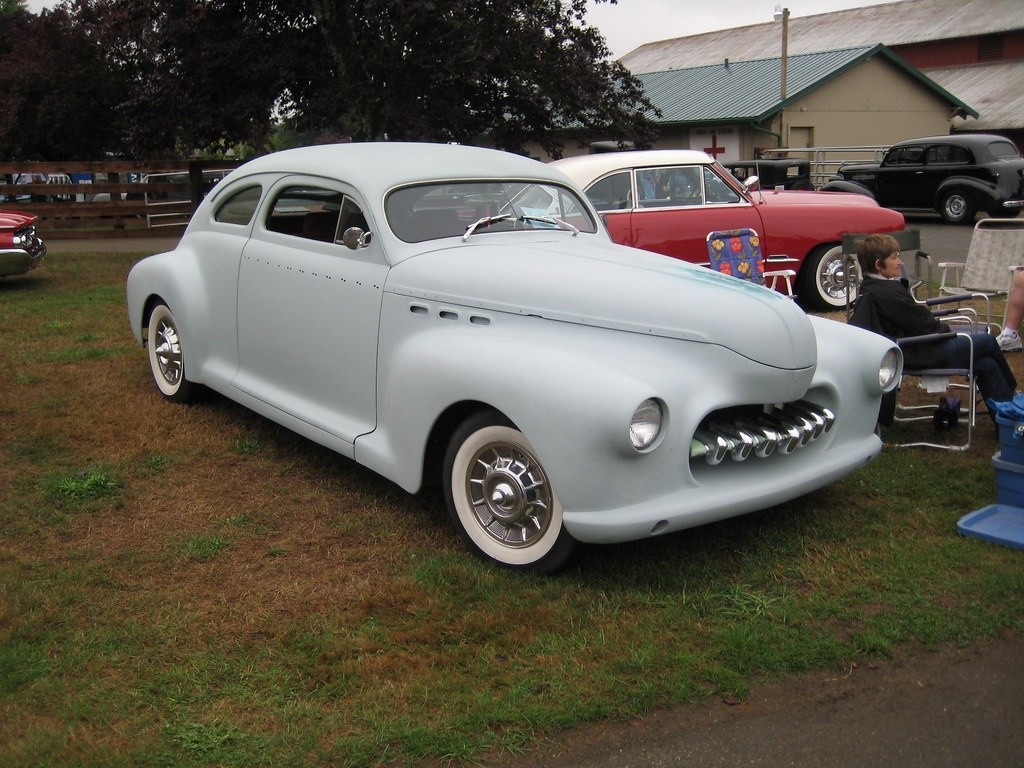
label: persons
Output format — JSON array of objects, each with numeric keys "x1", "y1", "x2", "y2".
[
  {"x1": 857, "y1": 234, "x2": 1020, "y2": 436},
  {"x1": 995, "y1": 270, "x2": 1024, "y2": 352}
]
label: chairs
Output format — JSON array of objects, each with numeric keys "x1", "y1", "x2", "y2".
[
  {"x1": 706, "y1": 228, "x2": 797, "y2": 300},
  {"x1": 878, "y1": 315, "x2": 973, "y2": 451},
  {"x1": 938, "y1": 218, "x2": 1024, "y2": 350},
  {"x1": 841, "y1": 228, "x2": 933, "y2": 323}
]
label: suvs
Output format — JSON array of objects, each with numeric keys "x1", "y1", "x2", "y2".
[
  {"x1": 816, "y1": 133, "x2": 1024, "y2": 226},
  {"x1": 714, "y1": 157, "x2": 815, "y2": 191}
]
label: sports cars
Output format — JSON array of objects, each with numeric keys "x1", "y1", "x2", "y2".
[
  {"x1": 489, "y1": 147, "x2": 907, "y2": 314},
  {"x1": 127, "y1": 140, "x2": 905, "y2": 581}
]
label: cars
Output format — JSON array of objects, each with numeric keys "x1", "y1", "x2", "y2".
[{"x1": 0, "y1": 208, "x2": 48, "y2": 276}]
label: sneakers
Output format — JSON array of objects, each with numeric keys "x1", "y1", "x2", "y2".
[{"x1": 996, "y1": 330, "x2": 1022, "y2": 352}]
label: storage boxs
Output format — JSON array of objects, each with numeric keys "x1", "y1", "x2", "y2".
[
  {"x1": 991, "y1": 451, "x2": 1024, "y2": 508},
  {"x1": 994, "y1": 408, "x2": 1024, "y2": 465}
]
